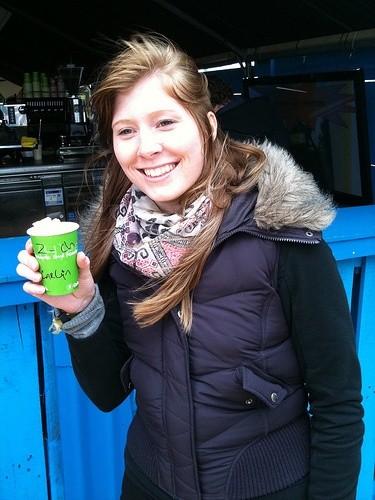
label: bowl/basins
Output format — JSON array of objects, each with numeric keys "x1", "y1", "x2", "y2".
[{"x1": 21, "y1": 151, "x2": 33, "y2": 157}]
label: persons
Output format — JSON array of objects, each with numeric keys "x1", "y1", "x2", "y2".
[
  {"x1": 16, "y1": 31, "x2": 365, "y2": 500},
  {"x1": 207, "y1": 75, "x2": 289, "y2": 150}
]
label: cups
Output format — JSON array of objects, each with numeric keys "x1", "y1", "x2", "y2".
[
  {"x1": 32, "y1": 140, "x2": 42, "y2": 161},
  {"x1": 27, "y1": 222, "x2": 81, "y2": 296},
  {"x1": 22, "y1": 72, "x2": 67, "y2": 98}
]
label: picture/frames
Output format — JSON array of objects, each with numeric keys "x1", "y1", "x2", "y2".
[{"x1": 242, "y1": 70, "x2": 371, "y2": 205}]
